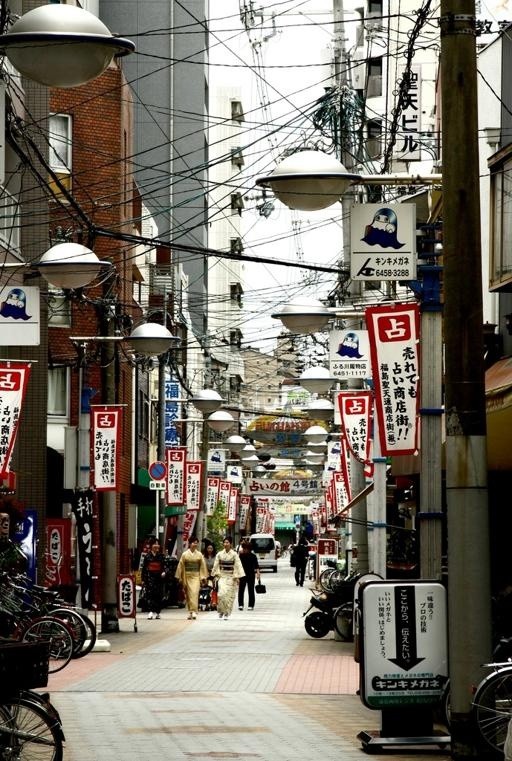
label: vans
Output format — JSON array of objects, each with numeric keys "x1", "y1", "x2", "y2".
[{"x1": 242, "y1": 534, "x2": 278, "y2": 572}]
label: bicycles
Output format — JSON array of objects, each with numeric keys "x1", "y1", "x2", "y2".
[
  {"x1": 0, "y1": 566, "x2": 102, "y2": 761},
  {"x1": 303, "y1": 565, "x2": 359, "y2": 642},
  {"x1": 440, "y1": 638, "x2": 512, "y2": 757}
]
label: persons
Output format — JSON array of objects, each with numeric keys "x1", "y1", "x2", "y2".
[
  {"x1": 202, "y1": 543, "x2": 216, "y2": 590},
  {"x1": 136, "y1": 537, "x2": 170, "y2": 620},
  {"x1": 237, "y1": 541, "x2": 267, "y2": 612},
  {"x1": 289, "y1": 539, "x2": 309, "y2": 587},
  {"x1": 210, "y1": 537, "x2": 246, "y2": 621},
  {"x1": 173, "y1": 537, "x2": 210, "y2": 620}
]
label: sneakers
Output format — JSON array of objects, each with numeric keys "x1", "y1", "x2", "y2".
[
  {"x1": 239, "y1": 605, "x2": 244, "y2": 610},
  {"x1": 248, "y1": 606, "x2": 254, "y2": 610},
  {"x1": 218, "y1": 612, "x2": 222, "y2": 618}
]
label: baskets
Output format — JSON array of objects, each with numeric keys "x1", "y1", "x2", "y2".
[{"x1": 0, "y1": 641, "x2": 51, "y2": 690}]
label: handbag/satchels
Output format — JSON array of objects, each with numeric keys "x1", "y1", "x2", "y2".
[
  {"x1": 256, "y1": 584, "x2": 266, "y2": 593},
  {"x1": 137, "y1": 594, "x2": 153, "y2": 607}
]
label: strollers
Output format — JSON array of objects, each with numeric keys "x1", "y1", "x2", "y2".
[{"x1": 199, "y1": 569, "x2": 220, "y2": 610}]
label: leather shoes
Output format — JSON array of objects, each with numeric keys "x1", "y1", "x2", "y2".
[
  {"x1": 147, "y1": 613, "x2": 154, "y2": 618},
  {"x1": 192, "y1": 612, "x2": 197, "y2": 618},
  {"x1": 187, "y1": 614, "x2": 192, "y2": 619},
  {"x1": 156, "y1": 615, "x2": 160, "y2": 618},
  {"x1": 224, "y1": 615, "x2": 229, "y2": 619}
]
